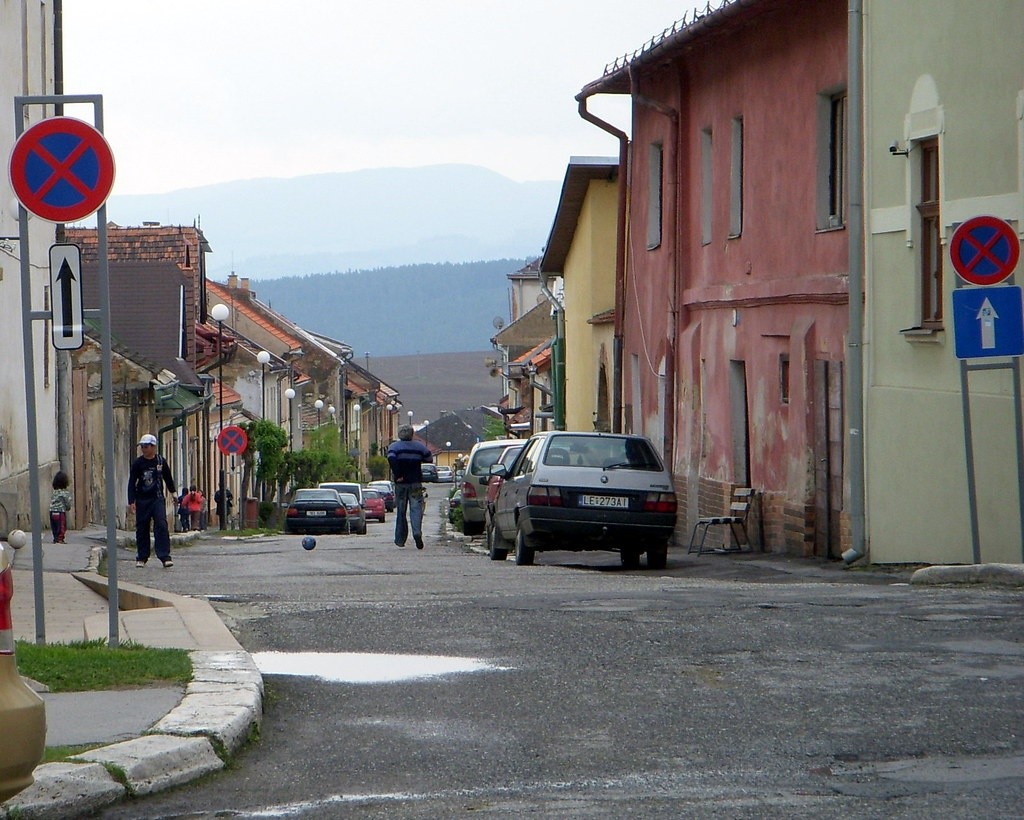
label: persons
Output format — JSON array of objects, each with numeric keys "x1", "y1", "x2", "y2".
[
  {"x1": 178, "y1": 485, "x2": 207, "y2": 532},
  {"x1": 49, "y1": 471, "x2": 73, "y2": 544},
  {"x1": 214, "y1": 483, "x2": 234, "y2": 531},
  {"x1": 387, "y1": 424, "x2": 433, "y2": 549},
  {"x1": 128, "y1": 434, "x2": 178, "y2": 567}
]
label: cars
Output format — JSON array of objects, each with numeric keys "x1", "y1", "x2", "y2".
[
  {"x1": 419, "y1": 463, "x2": 454, "y2": 483},
  {"x1": 478, "y1": 446, "x2": 528, "y2": 550},
  {"x1": 281, "y1": 489, "x2": 354, "y2": 535},
  {"x1": 490, "y1": 431, "x2": 679, "y2": 570},
  {"x1": 455, "y1": 439, "x2": 528, "y2": 536},
  {"x1": 361, "y1": 489, "x2": 387, "y2": 524},
  {"x1": 338, "y1": 493, "x2": 367, "y2": 535},
  {"x1": 367, "y1": 481, "x2": 397, "y2": 514}
]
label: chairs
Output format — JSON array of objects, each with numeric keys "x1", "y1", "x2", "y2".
[{"x1": 547, "y1": 446, "x2": 571, "y2": 465}]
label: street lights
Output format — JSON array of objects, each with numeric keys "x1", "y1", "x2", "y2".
[
  {"x1": 284, "y1": 389, "x2": 296, "y2": 487},
  {"x1": 257, "y1": 351, "x2": 270, "y2": 501},
  {"x1": 387, "y1": 404, "x2": 393, "y2": 480},
  {"x1": 446, "y1": 442, "x2": 451, "y2": 466},
  {"x1": 314, "y1": 400, "x2": 324, "y2": 455},
  {"x1": 407, "y1": 411, "x2": 413, "y2": 426},
  {"x1": 212, "y1": 303, "x2": 230, "y2": 529},
  {"x1": 424, "y1": 420, "x2": 429, "y2": 447},
  {"x1": 354, "y1": 404, "x2": 360, "y2": 482}
]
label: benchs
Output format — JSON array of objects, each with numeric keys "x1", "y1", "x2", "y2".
[{"x1": 687, "y1": 482, "x2": 761, "y2": 558}]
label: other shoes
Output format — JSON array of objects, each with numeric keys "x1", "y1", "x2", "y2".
[
  {"x1": 414, "y1": 534, "x2": 424, "y2": 549},
  {"x1": 394, "y1": 539, "x2": 405, "y2": 547},
  {"x1": 136, "y1": 560, "x2": 145, "y2": 567},
  {"x1": 164, "y1": 560, "x2": 173, "y2": 567}
]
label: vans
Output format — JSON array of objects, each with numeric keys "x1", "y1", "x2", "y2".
[{"x1": 317, "y1": 481, "x2": 365, "y2": 508}]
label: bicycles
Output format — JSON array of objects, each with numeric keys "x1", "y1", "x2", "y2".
[{"x1": 421, "y1": 486, "x2": 428, "y2": 513}]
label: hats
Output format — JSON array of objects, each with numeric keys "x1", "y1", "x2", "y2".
[{"x1": 136, "y1": 434, "x2": 157, "y2": 447}]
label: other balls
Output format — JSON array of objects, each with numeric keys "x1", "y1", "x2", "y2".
[{"x1": 301, "y1": 536, "x2": 316, "y2": 551}]
